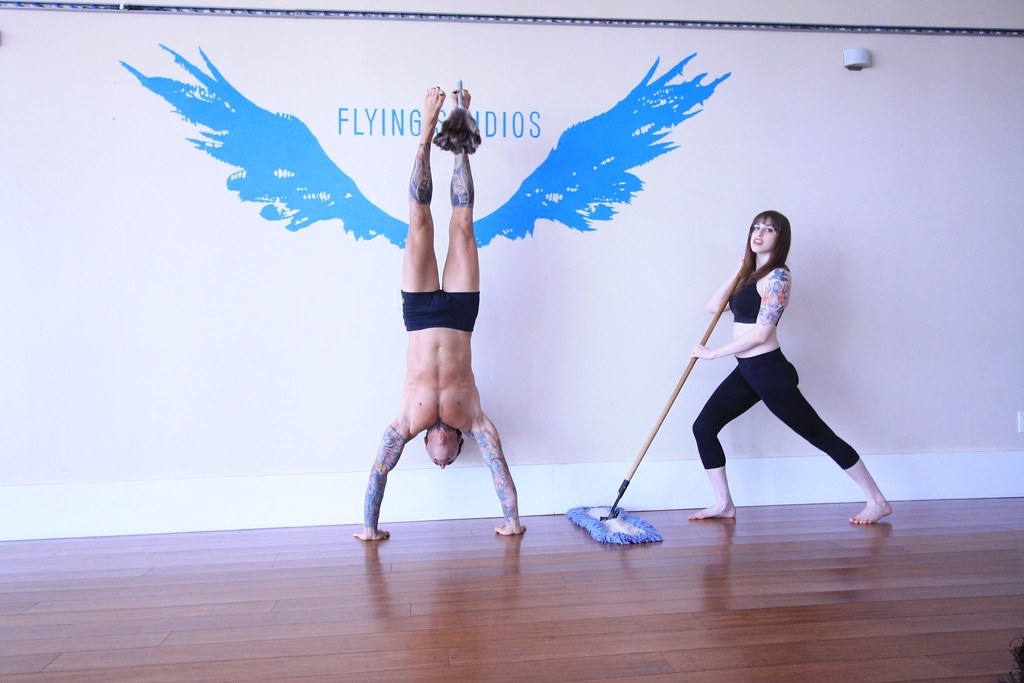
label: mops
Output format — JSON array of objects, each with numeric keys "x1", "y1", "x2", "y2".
[{"x1": 565, "y1": 262, "x2": 749, "y2": 547}]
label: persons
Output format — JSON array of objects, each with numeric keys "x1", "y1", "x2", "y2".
[
  {"x1": 352, "y1": 86, "x2": 527, "y2": 541},
  {"x1": 689, "y1": 210, "x2": 893, "y2": 526}
]
